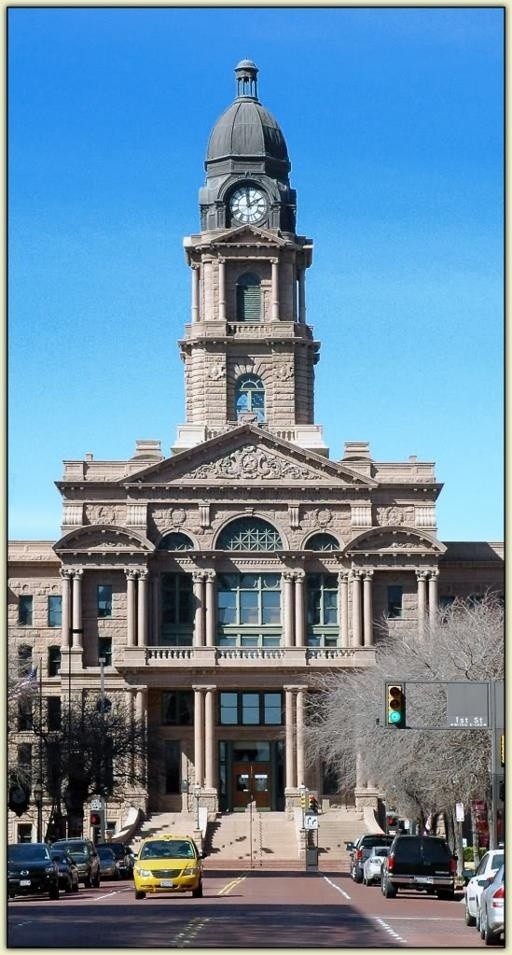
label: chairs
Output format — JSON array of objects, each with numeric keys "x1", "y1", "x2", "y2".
[{"x1": 149, "y1": 847, "x2": 159, "y2": 856}]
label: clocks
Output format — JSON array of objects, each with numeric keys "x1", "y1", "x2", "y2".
[{"x1": 229, "y1": 183, "x2": 268, "y2": 225}]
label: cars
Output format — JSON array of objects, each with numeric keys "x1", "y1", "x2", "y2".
[
  {"x1": 464, "y1": 842, "x2": 504, "y2": 945},
  {"x1": 8, "y1": 832, "x2": 207, "y2": 900},
  {"x1": 349, "y1": 833, "x2": 456, "y2": 900}
]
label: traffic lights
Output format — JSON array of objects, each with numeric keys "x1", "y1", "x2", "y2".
[
  {"x1": 300, "y1": 796, "x2": 315, "y2": 809},
  {"x1": 388, "y1": 686, "x2": 402, "y2": 724}
]
